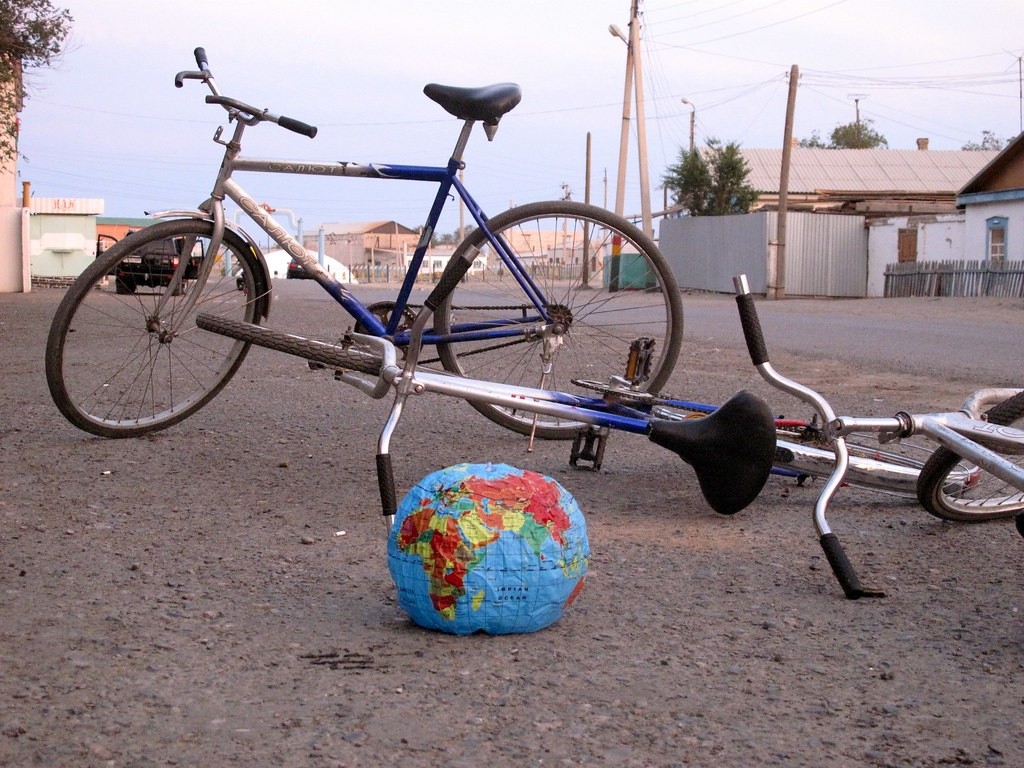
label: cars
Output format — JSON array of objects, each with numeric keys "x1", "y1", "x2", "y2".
[{"x1": 287, "y1": 257, "x2": 314, "y2": 279}]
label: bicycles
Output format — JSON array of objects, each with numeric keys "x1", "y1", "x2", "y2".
[
  {"x1": 45, "y1": 46, "x2": 686, "y2": 440},
  {"x1": 195, "y1": 313, "x2": 980, "y2": 542},
  {"x1": 730, "y1": 273, "x2": 1023, "y2": 599}
]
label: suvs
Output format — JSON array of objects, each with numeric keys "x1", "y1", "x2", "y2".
[{"x1": 96, "y1": 231, "x2": 205, "y2": 296}]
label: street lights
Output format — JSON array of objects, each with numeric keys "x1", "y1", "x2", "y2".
[
  {"x1": 681, "y1": 96, "x2": 695, "y2": 150},
  {"x1": 607, "y1": 25, "x2": 634, "y2": 292}
]
